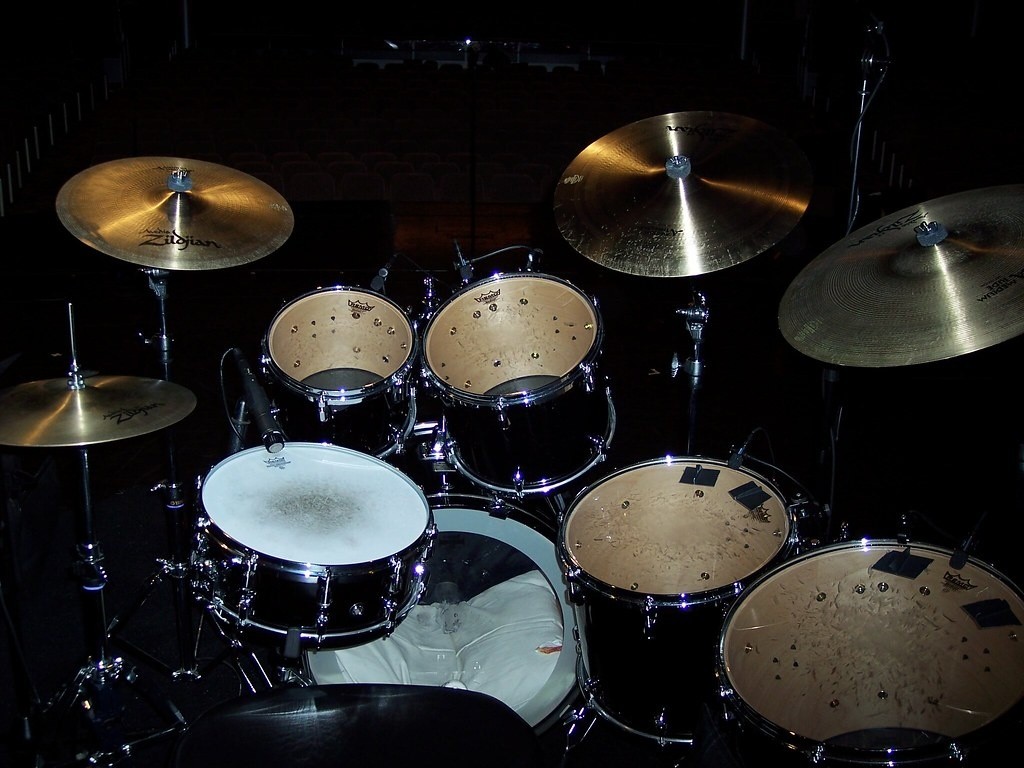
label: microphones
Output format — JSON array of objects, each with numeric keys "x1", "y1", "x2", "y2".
[{"x1": 233, "y1": 347, "x2": 284, "y2": 453}]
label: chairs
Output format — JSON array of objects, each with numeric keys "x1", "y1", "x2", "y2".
[{"x1": 59, "y1": 55, "x2": 1024, "y2": 227}]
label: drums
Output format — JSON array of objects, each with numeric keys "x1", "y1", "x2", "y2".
[
  {"x1": 422, "y1": 271, "x2": 617, "y2": 498},
  {"x1": 261, "y1": 284, "x2": 418, "y2": 460},
  {"x1": 184, "y1": 442, "x2": 437, "y2": 654},
  {"x1": 301, "y1": 419, "x2": 584, "y2": 734},
  {"x1": 554, "y1": 453, "x2": 807, "y2": 748},
  {"x1": 714, "y1": 536, "x2": 1024, "y2": 768}
]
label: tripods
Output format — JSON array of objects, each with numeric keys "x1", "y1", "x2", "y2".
[{"x1": 29, "y1": 265, "x2": 278, "y2": 739}]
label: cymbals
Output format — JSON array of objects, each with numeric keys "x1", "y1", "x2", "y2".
[
  {"x1": 777, "y1": 183, "x2": 1024, "y2": 369},
  {"x1": 54, "y1": 154, "x2": 294, "y2": 271},
  {"x1": 552, "y1": 110, "x2": 816, "y2": 279},
  {"x1": 0, "y1": 373, "x2": 198, "y2": 448}
]
